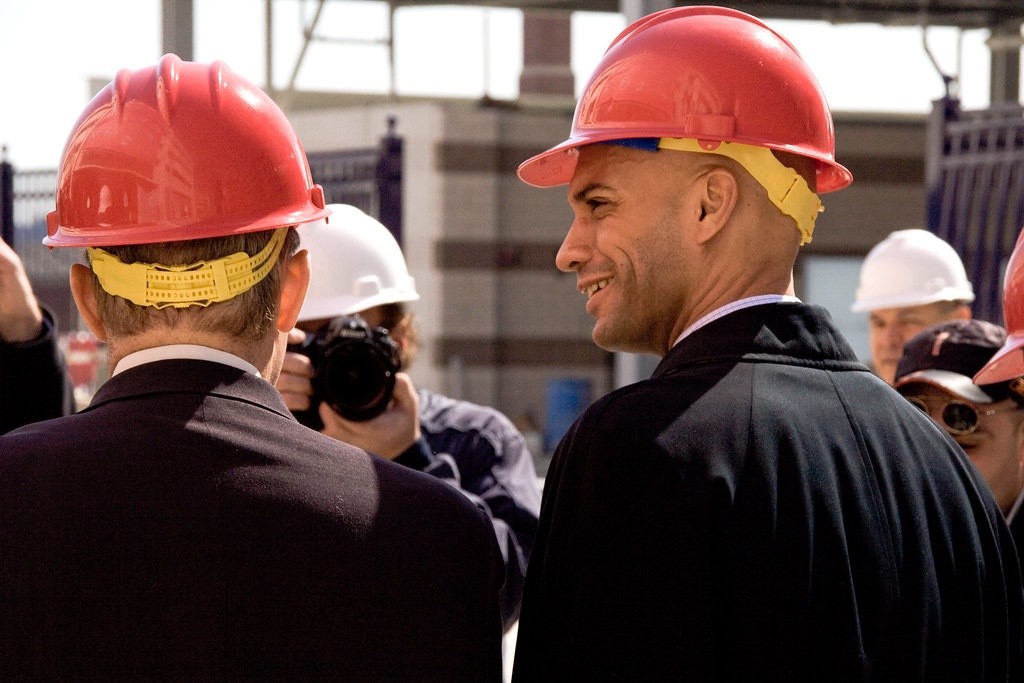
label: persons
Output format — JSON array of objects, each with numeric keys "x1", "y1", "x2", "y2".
[
  {"x1": 509, "y1": 5, "x2": 1024, "y2": 683},
  {"x1": 0, "y1": 54, "x2": 506, "y2": 683},
  {"x1": 0, "y1": 203, "x2": 1024, "y2": 683}
]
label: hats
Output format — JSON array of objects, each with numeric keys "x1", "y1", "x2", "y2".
[{"x1": 894, "y1": 318, "x2": 1016, "y2": 403}]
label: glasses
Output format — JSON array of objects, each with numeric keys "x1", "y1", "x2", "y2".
[{"x1": 906, "y1": 396, "x2": 1021, "y2": 436}]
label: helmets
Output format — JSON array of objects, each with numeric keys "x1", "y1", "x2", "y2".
[
  {"x1": 973, "y1": 226, "x2": 1024, "y2": 385},
  {"x1": 293, "y1": 203, "x2": 420, "y2": 322},
  {"x1": 516, "y1": 5, "x2": 853, "y2": 194},
  {"x1": 852, "y1": 229, "x2": 975, "y2": 313},
  {"x1": 43, "y1": 53, "x2": 331, "y2": 251}
]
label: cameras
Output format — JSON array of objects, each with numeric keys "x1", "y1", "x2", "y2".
[{"x1": 286, "y1": 313, "x2": 403, "y2": 433}]
